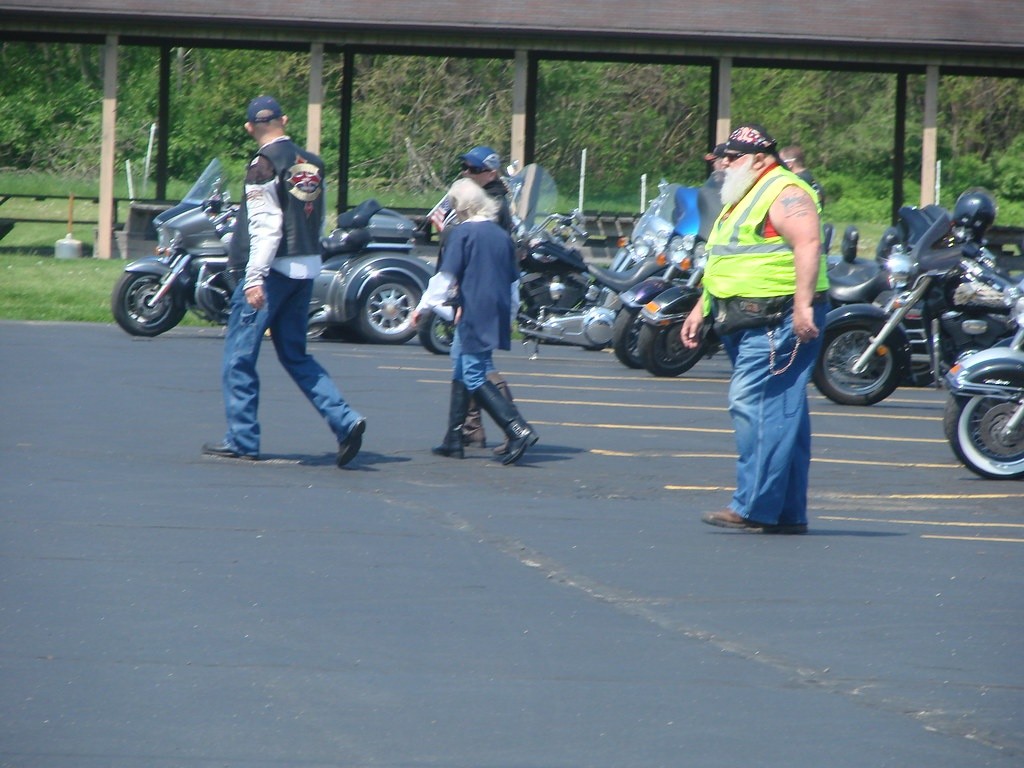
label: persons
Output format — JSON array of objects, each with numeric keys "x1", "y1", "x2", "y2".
[
  {"x1": 449, "y1": 146, "x2": 512, "y2": 455},
  {"x1": 410, "y1": 178, "x2": 539, "y2": 465},
  {"x1": 203, "y1": 95, "x2": 365, "y2": 467},
  {"x1": 705, "y1": 142, "x2": 824, "y2": 247},
  {"x1": 681, "y1": 123, "x2": 832, "y2": 532}
]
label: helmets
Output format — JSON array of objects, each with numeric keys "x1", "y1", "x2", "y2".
[{"x1": 954, "y1": 186, "x2": 996, "y2": 231}]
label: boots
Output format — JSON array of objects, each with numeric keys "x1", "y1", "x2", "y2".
[
  {"x1": 485, "y1": 372, "x2": 518, "y2": 455},
  {"x1": 471, "y1": 380, "x2": 539, "y2": 465},
  {"x1": 463, "y1": 398, "x2": 486, "y2": 448},
  {"x1": 431, "y1": 378, "x2": 486, "y2": 459}
]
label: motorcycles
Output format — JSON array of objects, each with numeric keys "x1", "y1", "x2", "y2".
[
  {"x1": 612, "y1": 171, "x2": 898, "y2": 377},
  {"x1": 418, "y1": 159, "x2": 643, "y2": 355},
  {"x1": 810, "y1": 212, "x2": 1024, "y2": 406},
  {"x1": 111, "y1": 158, "x2": 434, "y2": 346},
  {"x1": 944, "y1": 279, "x2": 1024, "y2": 481}
]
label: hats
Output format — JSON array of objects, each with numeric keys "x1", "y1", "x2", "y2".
[
  {"x1": 459, "y1": 147, "x2": 501, "y2": 170},
  {"x1": 460, "y1": 162, "x2": 492, "y2": 174},
  {"x1": 704, "y1": 142, "x2": 728, "y2": 161},
  {"x1": 726, "y1": 124, "x2": 777, "y2": 153},
  {"x1": 248, "y1": 95, "x2": 282, "y2": 123}
]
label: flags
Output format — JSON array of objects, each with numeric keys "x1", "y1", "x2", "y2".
[{"x1": 426, "y1": 193, "x2": 457, "y2": 232}]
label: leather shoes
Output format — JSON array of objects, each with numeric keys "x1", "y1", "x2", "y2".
[
  {"x1": 201, "y1": 441, "x2": 259, "y2": 461},
  {"x1": 700, "y1": 507, "x2": 808, "y2": 534},
  {"x1": 335, "y1": 420, "x2": 366, "y2": 468}
]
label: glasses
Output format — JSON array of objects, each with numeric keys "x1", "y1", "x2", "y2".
[
  {"x1": 709, "y1": 157, "x2": 721, "y2": 165},
  {"x1": 722, "y1": 151, "x2": 747, "y2": 163}
]
